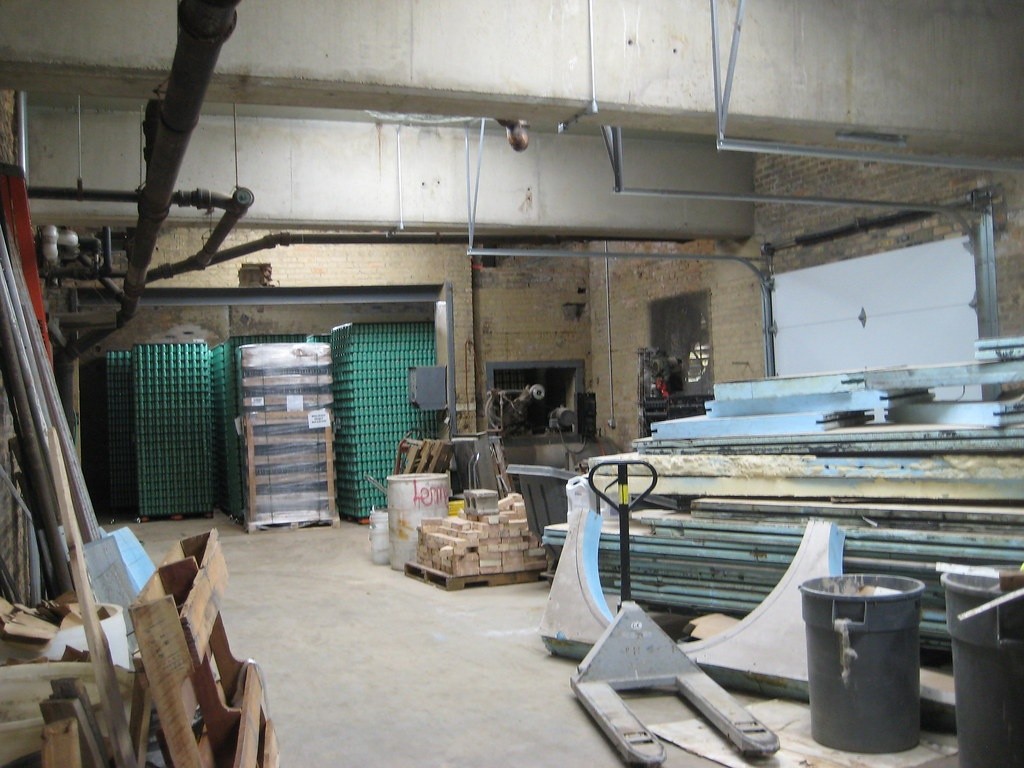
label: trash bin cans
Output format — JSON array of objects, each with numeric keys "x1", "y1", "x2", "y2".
[
  {"x1": 796, "y1": 571, "x2": 927, "y2": 755},
  {"x1": 940, "y1": 564, "x2": 1024, "y2": 768}
]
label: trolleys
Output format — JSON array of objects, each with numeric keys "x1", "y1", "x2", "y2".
[{"x1": 570, "y1": 459, "x2": 781, "y2": 768}]
